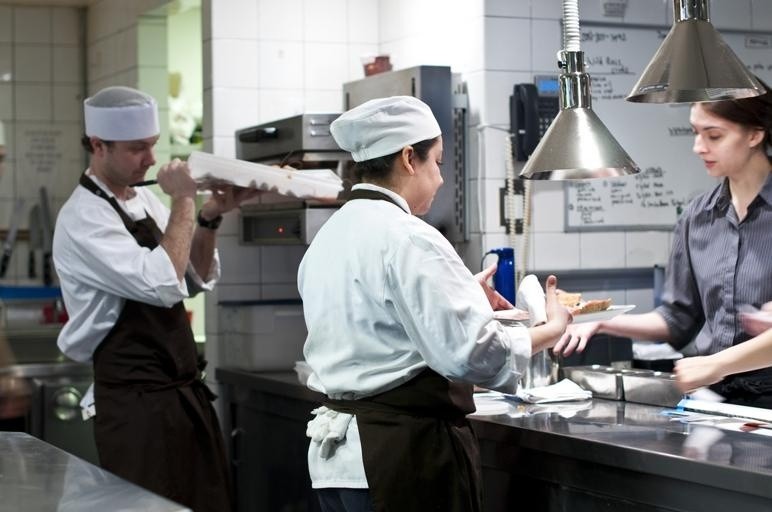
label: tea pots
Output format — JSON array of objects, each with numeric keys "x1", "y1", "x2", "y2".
[{"x1": 479, "y1": 246, "x2": 516, "y2": 309}]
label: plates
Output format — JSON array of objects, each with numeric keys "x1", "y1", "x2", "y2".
[{"x1": 491, "y1": 304, "x2": 636, "y2": 328}]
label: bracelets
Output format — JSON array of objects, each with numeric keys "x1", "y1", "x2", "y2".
[{"x1": 195, "y1": 206, "x2": 224, "y2": 233}]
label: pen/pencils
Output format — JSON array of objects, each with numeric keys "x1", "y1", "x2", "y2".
[{"x1": 130, "y1": 180, "x2": 159, "y2": 187}]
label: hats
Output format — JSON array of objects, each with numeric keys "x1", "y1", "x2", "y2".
[
  {"x1": 329, "y1": 95, "x2": 443, "y2": 163},
  {"x1": 83, "y1": 86, "x2": 160, "y2": 141}
]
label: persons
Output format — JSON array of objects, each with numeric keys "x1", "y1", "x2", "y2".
[
  {"x1": 671, "y1": 327, "x2": 771, "y2": 398},
  {"x1": 292, "y1": 93, "x2": 584, "y2": 512},
  {"x1": 50, "y1": 86, "x2": 298, "y2": 512},
  {"x1": 554, "y1": 71, "x2": 772, "y2": 411}
]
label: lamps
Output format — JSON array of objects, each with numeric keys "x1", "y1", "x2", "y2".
[
  {"x1": 624, "y1": 0, "x2": 767, "y2": 103},
  {"x1": 518, "y1": 0, "x2": 640, "y2": 180}
]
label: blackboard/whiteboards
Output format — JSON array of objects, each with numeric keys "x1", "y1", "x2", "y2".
[{"x1": 559, "y1": 19, "x2": 772, "y2": 234}]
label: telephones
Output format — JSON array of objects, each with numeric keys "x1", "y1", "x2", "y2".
[{"x1": 509, "y1": 75, "x2": 560, "y2": 162}]
